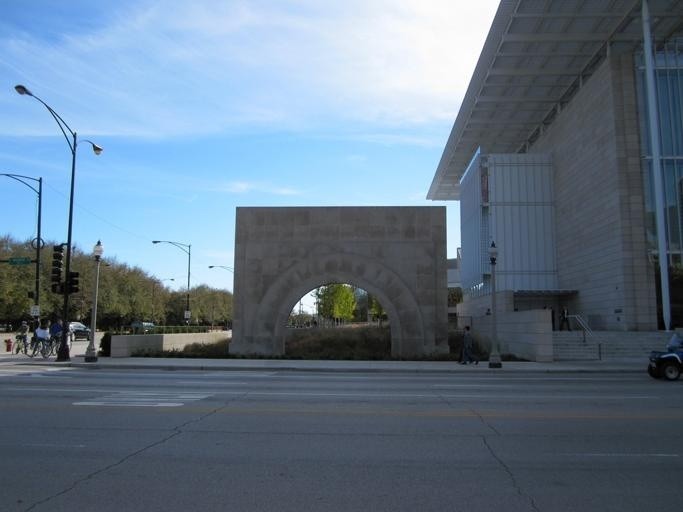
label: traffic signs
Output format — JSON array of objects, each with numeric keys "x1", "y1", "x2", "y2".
[{"x1": 9, "y1": 257, "x2": 31, "y2": 265}]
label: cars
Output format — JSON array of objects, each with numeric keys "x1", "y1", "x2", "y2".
[
  {"x1": 648, "y1": 351, "x2": 683, "y2": 380},
  {"x1": 67, "y1": 322, "x2": 91, "y2": 342}
]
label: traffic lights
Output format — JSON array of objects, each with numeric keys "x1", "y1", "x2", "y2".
[
  {"x1": 51, "y1": 246, "x2": 64, "y2": 282},
  {"x1": 67, "y1": 271, "x2": 80, "y2": 293}
]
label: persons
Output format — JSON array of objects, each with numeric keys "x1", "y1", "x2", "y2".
[
  {"x1": 667, "y1": 328, "x2": 683, "y2": 360},
  {"x1": 15, "y1": 322, "x2": 30, "y2": 354},
  {"x1": 458, "y1": 326, "x2": 480, "y2": 365},
  {"x1": 30, "y1": 319, "x2": 50, "y2": 356},
  {"x1": 486, "y1": 308, "x2": 492, "y2": 315},
  {"x1": 49, "y1": 319, "x2": 63, "y2": 345},
  {"x1": 543, "y1": 304, "x2": 572, "y2": 331}
]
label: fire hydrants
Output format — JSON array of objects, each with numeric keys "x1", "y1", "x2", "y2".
[{"x1": 5, "y1": 339, "x2": 12, "y2": 351}]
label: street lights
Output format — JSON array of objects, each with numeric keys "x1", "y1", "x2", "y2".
[
  {"x1": 210, "y1": 265, "x2": 233, "y2": 272},
  {"x1": 85, "y1": 241, "x2": 103, "y2": 362},
  {"x1": 153, "y1": 241, "x2": 190, "y2": 326},
  {"x1": 488, "y1": 241, "x2": 502, "y2": 368},
  {"x1": 14, "y1": 85, "x2": 102, "y2": 362}
]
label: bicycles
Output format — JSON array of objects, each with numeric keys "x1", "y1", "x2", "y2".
[
  {"x1": 12, "y1": 333, "x2": 31, "y2": 354},
  {"x1": 24, "y1": 336, "x2": 61, "y2": 358}
]
